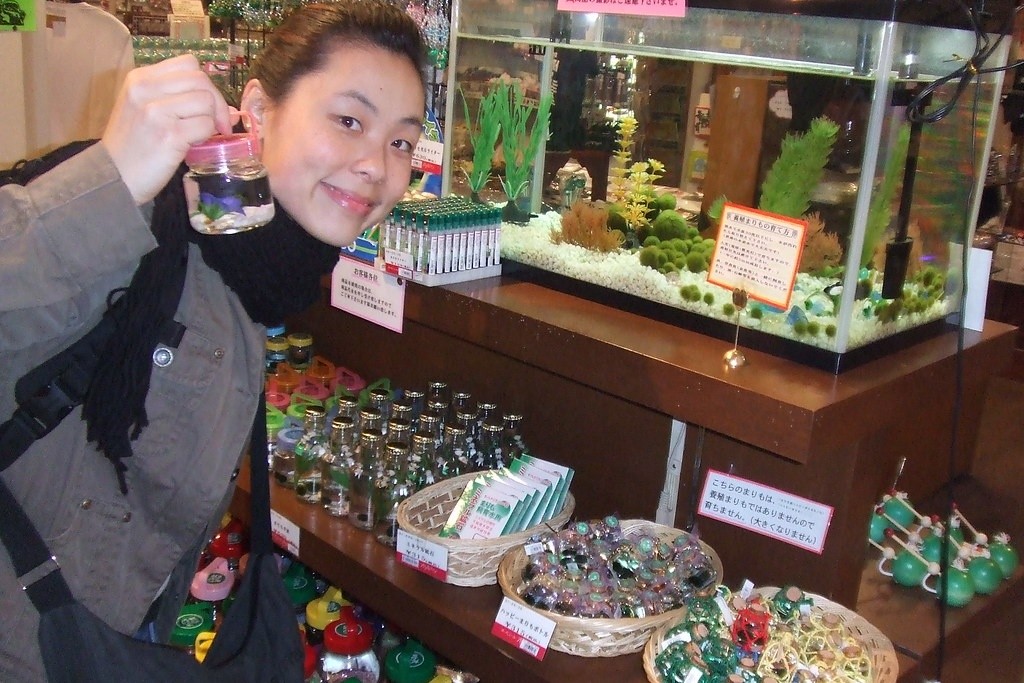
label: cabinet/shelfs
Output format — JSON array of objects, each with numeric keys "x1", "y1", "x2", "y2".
[
  {"x1": 233, "y1": 276, "x2": 1024, "y2": 683},
  {"x1": 700, "y1": 76, "x2": 794, "y2": 211}
]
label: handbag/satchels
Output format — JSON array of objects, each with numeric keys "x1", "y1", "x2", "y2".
[{"x1": 36, "y1": 550, "x2": 306, "y2": 683}]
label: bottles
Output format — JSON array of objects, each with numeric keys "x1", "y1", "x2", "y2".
[
  {"x1": 171, "y1": 326, "x2": 867, "y2": 683},
  {"x1": 378, "y1": 195, "x2": 502, "y2": 274},
  {"x1": 184, "y1": 133, "x2": 277, "y2": 234}
]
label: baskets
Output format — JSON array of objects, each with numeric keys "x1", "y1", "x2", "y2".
[
  {"x1": 397, "y1": 470, "x2": 576, "y2": 587},
  {"x1": 642, "y1": 587, "x2": 899, "y2": 683},
  {"x1": 496, "y1": 518, "x2": 723, "y2": 657}
]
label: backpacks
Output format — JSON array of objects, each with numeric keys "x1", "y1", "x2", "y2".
[{"x1": 0, "y1": 139, "x2": 187, "y2": 498}]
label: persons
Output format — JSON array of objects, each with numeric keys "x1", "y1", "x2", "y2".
[{"x1": 0, "y1": 0, "x2": 429, "y2": 682}]
